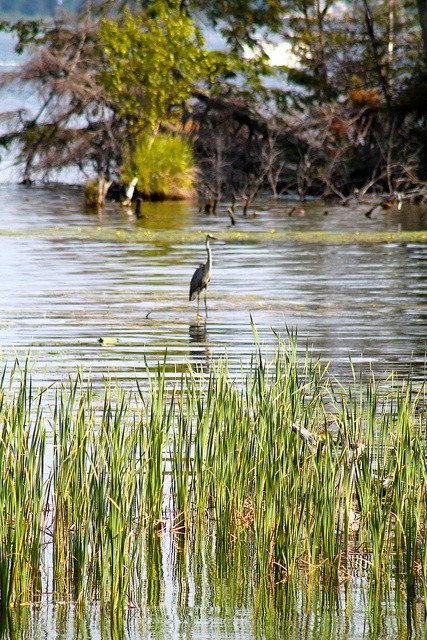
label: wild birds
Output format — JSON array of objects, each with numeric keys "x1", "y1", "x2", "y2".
[{"x1": 190, "y1": 234, "x2": 219, "y2": 313}]
